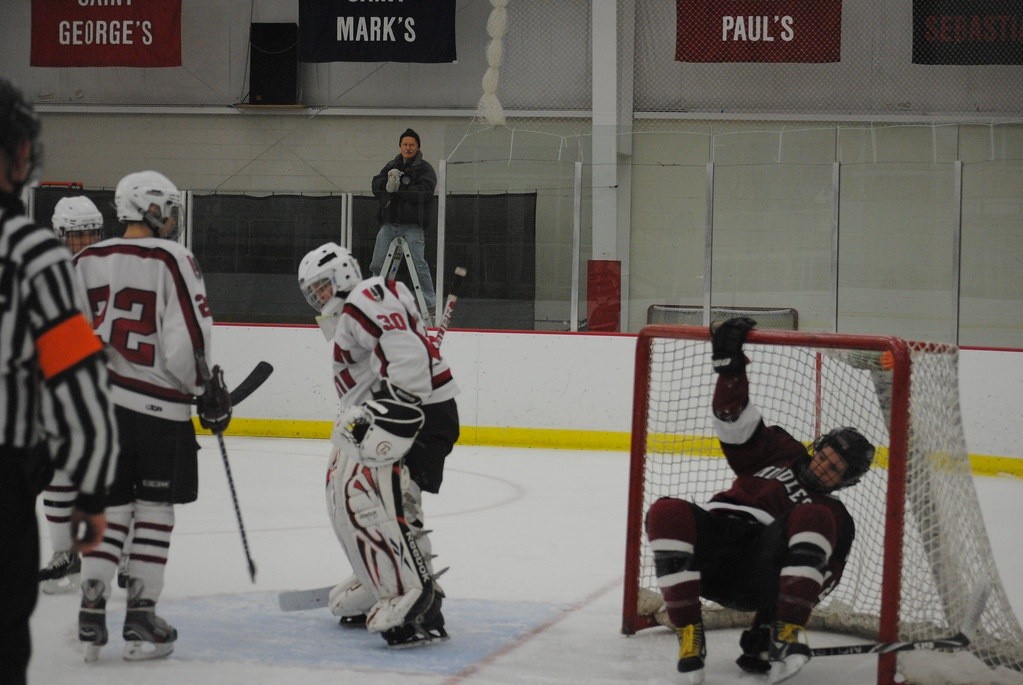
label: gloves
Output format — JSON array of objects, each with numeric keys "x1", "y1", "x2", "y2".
[
  {"x1": 385, "y1": 177, "x2": 398, "y2": 192},
  {"x1": 196, "y1": 370, "x2": 231, "y2": 433},
  {"x1": 387, "y1": 169, "x2": 403, "y2": 178},
  {"x1": 709, "y1": 316, "x2": 757, "y2": 373},
  {"x1": 734, "y1": 627, "x2": 770, "y2": 671}
]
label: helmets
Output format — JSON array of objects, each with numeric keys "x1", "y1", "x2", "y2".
[
  {"x1": 298, "y1": 241, "x2": 363, "y2": 342},
  {"x1": 0, "y1": 77, "x2": 39, "y2": 148},
  {"x1": 797, "y1": 426, "x2": 875, "y2": 495},
  {"x1": 51, "y1": 196, "x2": 103, "y2": 238},
  {"x1": 114, "y1": 170, "x2": 182, "y2": 221}
]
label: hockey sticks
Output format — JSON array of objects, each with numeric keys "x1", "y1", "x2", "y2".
[
  {"x1": 195, "y1": 349, "x2": 256, "y2": 583},
  {"x1": 190, "y1": 360, "x2": 273, "y2": 408},
  {"x1": 810, "y1": 560, "x2": 991, "y2": 657},
  {"x1": 278, "y1": 266, "x2": 468, "y2": 611}
]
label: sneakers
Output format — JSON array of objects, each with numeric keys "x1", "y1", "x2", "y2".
[
  {"x1": 339, "y1": 615, "x2": 368, "y2": 629},
  {"x1": 676, "y1": 620, "x2": 707, "y2": 685},
  {"x1": 117, "y1": 555, "x2": 132, "y2": 587},
  {"x1": 765, "y1": 623, "x2": 811, "y2": 685},
  {"x1": 121, "y1": 579, "x2": 177, "y2": 660},
  {"x1": 380, "y1": 607, "x2": 449, "y2": 649},
  {"x1": 39, "y1": 550, "x2": 82, "y2": 594},
  {"x1": 78, "y1": 577, "x2": 108, "y2": 663}
]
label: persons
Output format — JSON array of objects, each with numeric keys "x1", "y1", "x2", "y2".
[
  {"x1": 297, "y1": 243, "x2": 460, "y2": 651},
  {"x1": 71, "y1": 170, "x2": 233, "y2": 663},
  {"x1": 40, "y1": 194, "x2": 105, "y2": 595},
  {"x1": 646, "y1": 317, "x2": 876, "y2": 685},
  {"x1": 368, "y1": 128, "x2": 436, "y2": 326},
  {"x1": 0, "y1": 75, "x2": 121, "y2": 684}
]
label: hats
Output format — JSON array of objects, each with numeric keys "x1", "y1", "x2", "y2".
[{"x1": 398, "y1": 128, "x2": 420, "y2": 149}]
